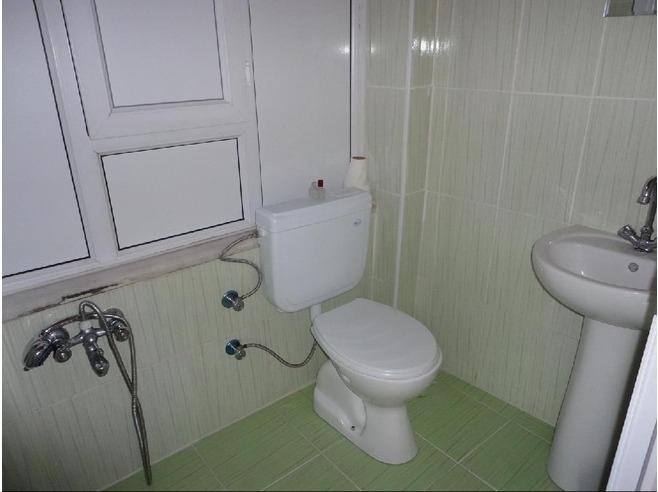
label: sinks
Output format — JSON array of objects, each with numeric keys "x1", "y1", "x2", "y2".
[{"x1": 531, "y1": 225, "x2": 657, "y2": 329}]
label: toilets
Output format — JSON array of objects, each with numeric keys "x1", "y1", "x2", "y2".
[{"x1": 255, "y1": 187, "x2": 443, "y2": 465}]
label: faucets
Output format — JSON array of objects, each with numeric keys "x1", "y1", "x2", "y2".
[{"x1": 637, "y1": 176, "x2": 657, "y2": 239}]
